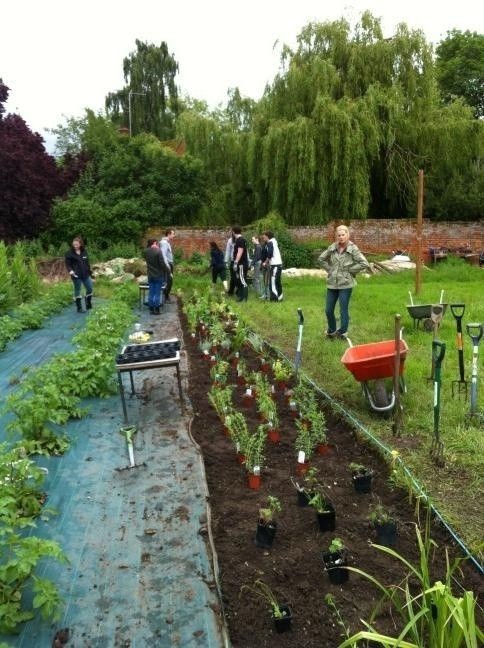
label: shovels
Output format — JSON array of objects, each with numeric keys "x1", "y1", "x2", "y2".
[{"x1": 426, "y1": 305, "x2": 442, "y2": 387}]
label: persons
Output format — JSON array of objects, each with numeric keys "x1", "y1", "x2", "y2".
[
  {"x1": 231, "y1": 227, "x2": 249, "y2": 303},
  {"x1": 248, "y1": 235, "x2": 264, "y2": 296},
  {"x1": 258, "y1": 232, "x2": 270, "y2": 300},
  {"x1": 316, "y1": 224, "x2": 368, "y2": 337},
  {"x1": 261, "y1": 231, "x2": 285, "y2": 302},
  {"x1": 224, "y1": 231, "x2": 251, "y2": 294},
  {"x1": 140, "y1": 238, "x2": 173, "y2": 315},
  {"x1": 157, "y1": 228, "x2": 175, "y2": 305},
  {"x1": 206, "y1": 241, "x2": 229, "y2": 293},
  {"x1": 65, "y1": 236, "x2": 97, "y2": 315}
]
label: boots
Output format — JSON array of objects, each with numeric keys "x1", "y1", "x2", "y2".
[
  {"x1": 77, "y1": 297, "x2": 85, "y2": 312},
  {"x1": 86, "y1": 295, "x2": 92, "y2": 310},
  {"x1": 223, "y1": 280, "x2": 228, "y2": 290},
  {"x1": 150, "y1": 306, "x2": 163, "y2": 315}
]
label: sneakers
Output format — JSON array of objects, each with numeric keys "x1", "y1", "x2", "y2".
[
  {"x1": 327, "y1": 329, "x2": 335, "y2": 335},
  {"x1": 338, "y1": 330, "x2": 346, "y2": 335},
  {"x1": 258, "y1": 293, "x2": 265, "y2": 298}
]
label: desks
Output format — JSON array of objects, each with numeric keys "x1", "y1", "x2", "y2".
[
  {"x1": 139, "y1": 282, "x2": 167, "y2": 311},
  {"x1": 114, "y1": 338, "x2": 186, "y2": 425}
]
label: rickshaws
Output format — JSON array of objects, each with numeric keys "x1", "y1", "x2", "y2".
[
  {"x1": 340, "y1": 325, "x2": 410, "y2": 420},
  {"x1": 406, "y1": 289, "x2": 449, "y2": 332}
]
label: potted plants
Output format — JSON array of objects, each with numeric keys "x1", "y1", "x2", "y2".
[
  {"x1": 249, "y1": 456, "x2": 411, "y2": 634},
  {"x1": 176, "y1": 285, "x2": 333, "y2": 487}
]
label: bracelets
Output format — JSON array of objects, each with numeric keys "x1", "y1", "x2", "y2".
[{"x1": 233, "y1": 260, "x2": 239, "y2": 264}]
